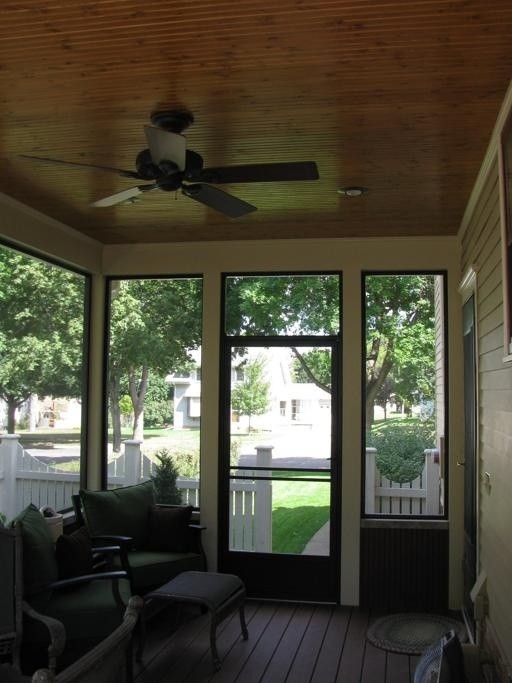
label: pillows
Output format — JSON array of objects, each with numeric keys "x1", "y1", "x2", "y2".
[
  {"x1": 80, "y1": 481, "x2": 156, "y2": 553},
  {"x1": 144, "y1": 505, "x2": 193, "y2": 551},
  {"x1": 4, "y1": 503, "x2": 57, "y2": 602},
  {"x1": 56, "y1": 527, "x2": 93, "y2": 586}
]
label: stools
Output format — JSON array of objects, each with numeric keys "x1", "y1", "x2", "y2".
[{"x1": 136, "y1": 571, "x2": 248, "y2": 671}]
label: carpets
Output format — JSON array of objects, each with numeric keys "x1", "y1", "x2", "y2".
[{"x1": 367, "y1": 614, "x2": 468, "y2": 655}]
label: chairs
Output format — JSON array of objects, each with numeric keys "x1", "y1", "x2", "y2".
[
  {"x1": 32, "y1": 596, "x2": 143, "y2": 683},
  {"x1": 72, "y1": 494, "x2": 209, "y2": 630},
  {"x1": 1, "y1": 520, "x2": 66, "y2": 683},
  {"x1": 25, "y1": 546, "x2": 141, "y2": 683}
]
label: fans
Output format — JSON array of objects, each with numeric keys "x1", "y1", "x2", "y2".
[{"x1": 18, "y1": 109, "x2": 318, "y2": 219}]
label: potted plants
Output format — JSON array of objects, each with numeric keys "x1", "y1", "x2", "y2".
[{"x1": 40, "y1": 504, "x2": 63, "y2": 543}]
